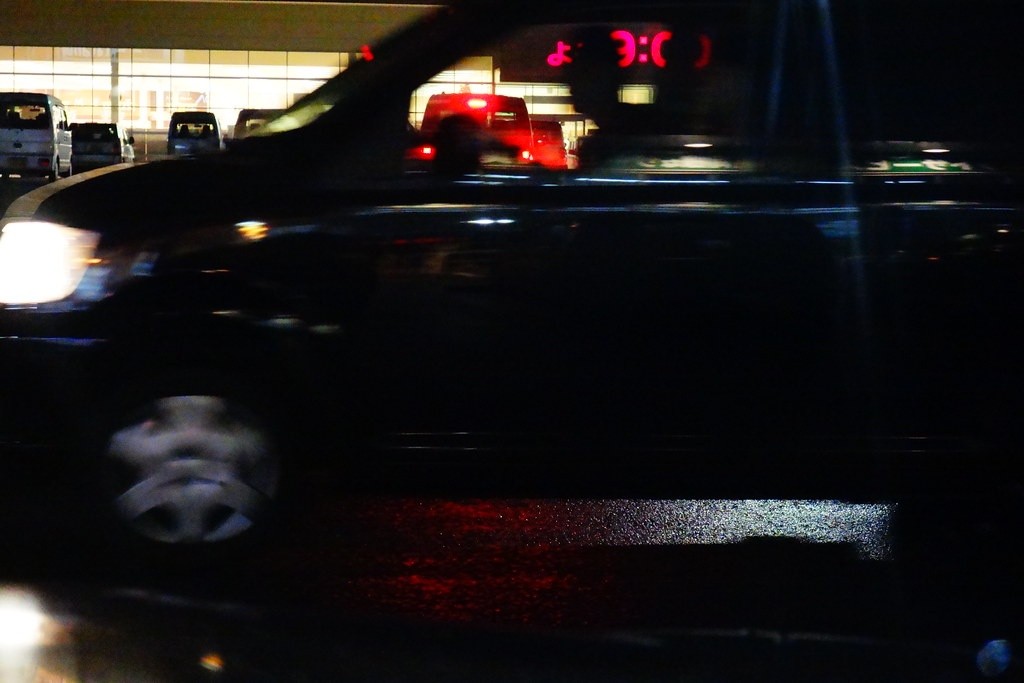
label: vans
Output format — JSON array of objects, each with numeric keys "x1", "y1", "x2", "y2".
[
  {"x1": 0, "y1": 91, "x2": 72, "y2": 185},
  {"x1": 69, "y1": 123, "x2": 124, "y2": 179},
  {"x1": 166, "y1": 112, "x2": 221, "y2": 154},
  {"x1": 230, "y1": 108, "x2": 290, "y2": 148}
]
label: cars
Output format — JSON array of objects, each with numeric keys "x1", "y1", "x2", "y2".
[{"x1": 0, "y1": 0, "x2": 1024, "y2": 649}]
